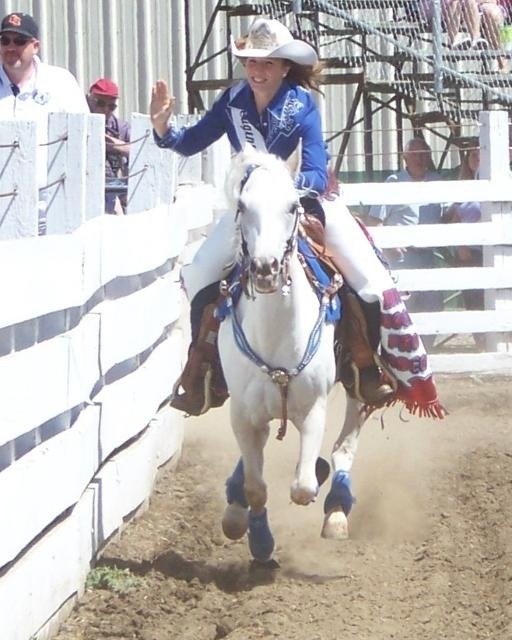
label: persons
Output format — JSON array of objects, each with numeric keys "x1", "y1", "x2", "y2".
[
  {"x1": 0, "y1": 12, "x2": 91, "y2": 115},
  {"x1": 366, "y1": 138, "x2": 445, "y2": 345},
  {"x1": 86, "y1": 78, "x2": 132, "y2": 214},
  {"x1": 447, "y1": 142, "x2": 486, "y2": 350},
  {"x1": 417, "y1": 0, "x2": 512, "y2": 74},
  {"x1": 149, "y1": 20, "x2": 393, "y2": 405}
]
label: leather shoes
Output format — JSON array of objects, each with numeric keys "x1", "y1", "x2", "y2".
[{"x1": 348, "y1": 378, "x2": 395, "y2": 402}]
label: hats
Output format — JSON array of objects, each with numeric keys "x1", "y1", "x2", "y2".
[
  {"x1": 230, "y1": 18, "x2": 318, "y2": 70},
  {"x1": 89, "y1": 78, "x2": 120, "y2": 98},
  {"x1": 0, "y1": 12, "x2": 38, "y2": 41}
]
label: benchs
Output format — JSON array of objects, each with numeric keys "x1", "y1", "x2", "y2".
[{"x1": 240, "y1": 0, "x2": 512, "y2": 150}]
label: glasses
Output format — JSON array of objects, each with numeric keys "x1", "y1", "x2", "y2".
[
  {"x1": 94, "y1": 98, "x2": 117, "y2": 111},
  {"x1": 0, "y1": 35, "x2": 35, "y2": 45}
]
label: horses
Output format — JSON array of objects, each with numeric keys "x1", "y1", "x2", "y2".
[{"x1": 217, "y1": 136, "x2": 377, "y2": 565}]
